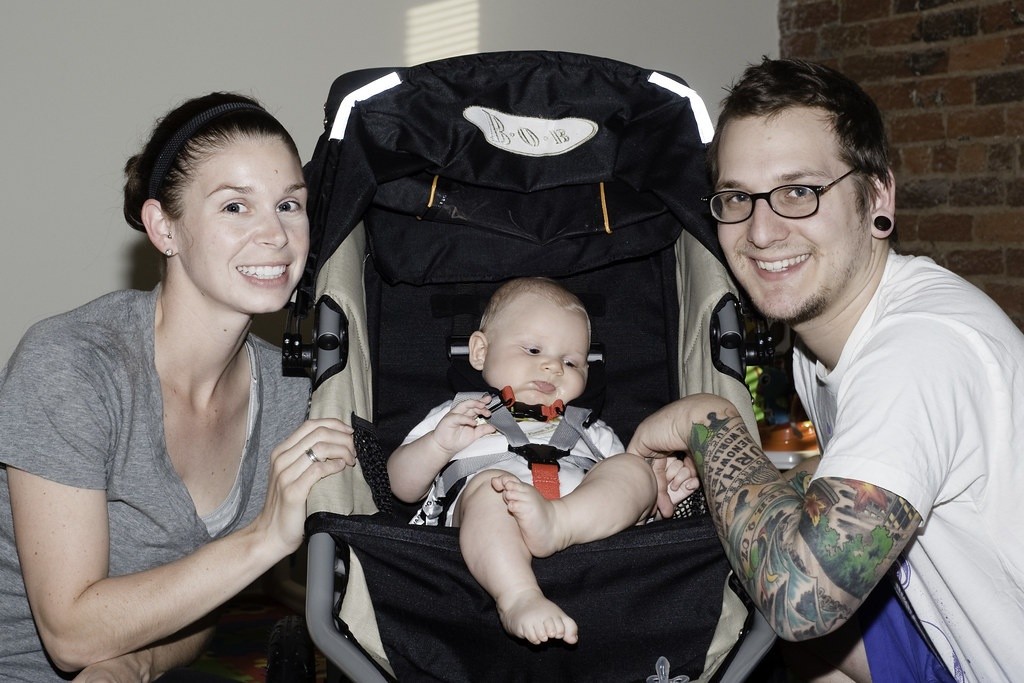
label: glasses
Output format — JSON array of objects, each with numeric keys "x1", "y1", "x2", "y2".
[{"x1": 701, "y1": 167, "x2": 857, "y2": 224}]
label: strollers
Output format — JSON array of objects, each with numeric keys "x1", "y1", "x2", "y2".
[{"x1": 283, "y1": 50, "x2": 777, "y2": 683}]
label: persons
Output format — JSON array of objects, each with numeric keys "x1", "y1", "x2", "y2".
[
  {"x1": 387, "y1": 276, "x2": 699, "y2": 644},
  {"x1": 627, "y1": 51, "x2": 1024, "y2": 683},
  {"x1": 0, "y1": 90, "x2": 360, "y2": 683}
]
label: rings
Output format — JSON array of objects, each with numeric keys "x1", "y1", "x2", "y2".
[{"x1": 305, "y1": 448, "x2": 320, "y2": 463}]
